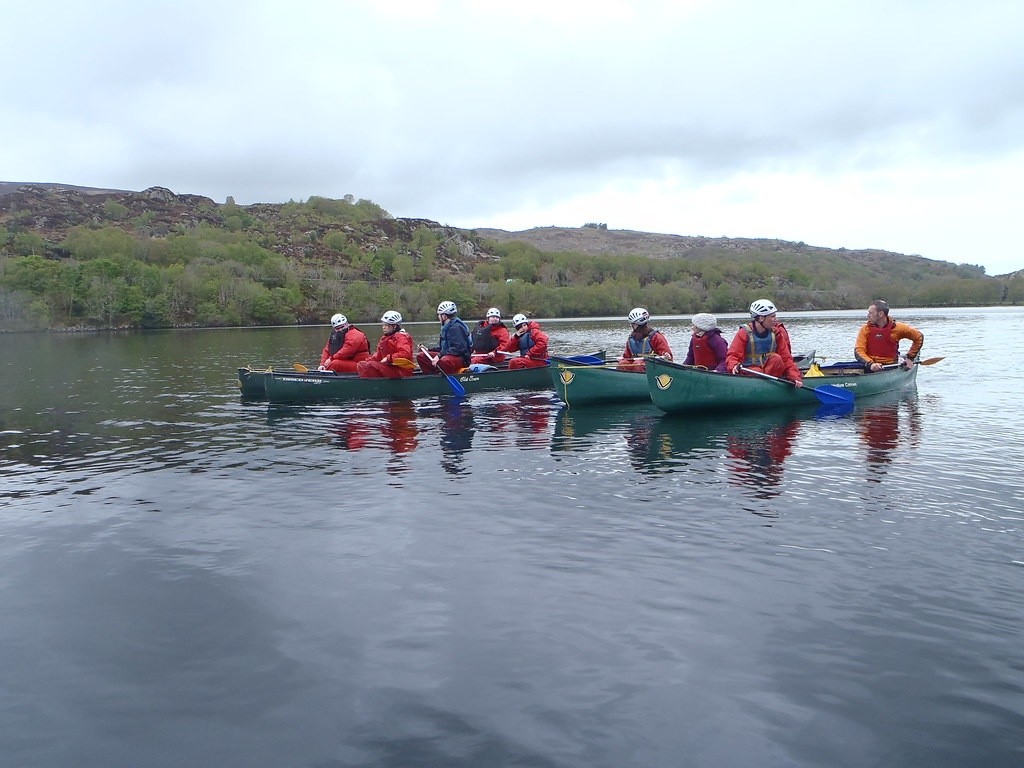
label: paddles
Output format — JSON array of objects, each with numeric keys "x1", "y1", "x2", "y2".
[
  {"x1": 736, "y1": 361, "x2": 856, "y2": 404},
  {"x1": 385, "y1": 357, "x2": 416, "y2": 369},
  {"x1": 294, "y1": 364, "x2": 338, "y2": 376},
  {"x1": 495, "y1": 348, "x2": 551, "y2": 363},
  {"x1": 880, "y1": 356, "x2": 947, "y2": 370},
  {"x1": 566, "y1": 353, "x2": 669, "y2": 366},
  {"x1": 419, "y1": 343, "x2": 465, "y2": 395},
  {"x1": 471, "y1": 352, "x2": 494, "y2": 357}
]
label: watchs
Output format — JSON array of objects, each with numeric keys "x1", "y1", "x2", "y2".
[{"x1": 867, "y1": 362, "x2": 873, "y2": 370}]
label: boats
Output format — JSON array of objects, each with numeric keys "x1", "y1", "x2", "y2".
[
  {"x1": 549, "y1": 349, "x2": 819, "y2": 411},
  {"x1": 236, "y1": 350, "x2": 607, "y2": 408},
  {"x1": 641, "y1": 350, "x2": 922, "y2": 416}
]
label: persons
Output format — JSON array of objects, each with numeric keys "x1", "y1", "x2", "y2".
[
  {"x1": 854, "y1": 300, "x2": 924, "y2": 372},
  {"x1": 616, "y1": 308, "x2": 673, "y2": 373},
  {"x1": 416, "y1": 301, "x2": 474, "y2": 375},
  {"x1": 320, "y1": 313, "x2": 371, "y2": 373},
  {"x1": 505, "y1": 314, "x2": 550, "y2": 370},
  {"x1": 356, "y1": 310, "x2": 414, "y2": 378},
  {"x1": 683, "y1": 313, "x2": 728, "y2": 372},
  {"x1": 470, "y1": 307, "x2": 509, "y2": 367},
  {"x1": 725, "y1": 299, "x2": 802, "y2": 389}
]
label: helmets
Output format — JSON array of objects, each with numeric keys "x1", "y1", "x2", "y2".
[
  {"x1": 749, "y1": 299, "x2": 777, "y2": 318},
  {"x1": 437, "y1": 301, "x2": 457, "y2": 314},
  {"x1": 486, "y1": 308, "x2": 500, "y2": 318},
  {"x1": 381, "y1": 311, "x2": 402, "y2": 324},
  {"x1": 330, "y1": 314, "x2": 347, "y2": 328},
  {"x1": 628, "y1": 308, "x2": 650, "y2": 325},
  {"x1": 512, "y1": 313, "x2": 528, "y2": 327}
]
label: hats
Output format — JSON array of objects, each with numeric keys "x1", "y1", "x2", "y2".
[{"x1": 692, "y1": 312, "x2": 717, "y2": 331}]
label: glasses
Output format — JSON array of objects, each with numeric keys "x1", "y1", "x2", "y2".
[
  {"x1": 382, "y1": 323, "x2": 392, "y2": 327},
  {"x1": 334, "y1": 326, "x2": 344, "y2": 330}
]
